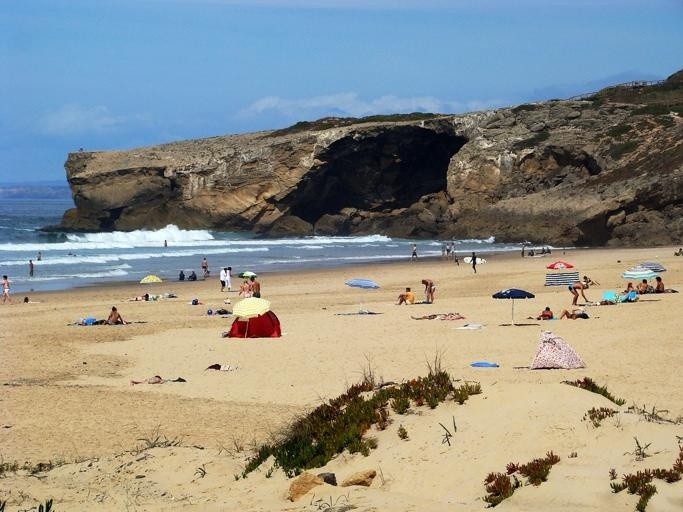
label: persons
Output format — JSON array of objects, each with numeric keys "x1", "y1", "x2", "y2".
[
  {"x1": 1, "y1": 239, "x2": 280, "y2": 338},
  {"x1": 130, "y1": 374, "x2": 166, "y2": 387},
  {"x1": 395, "y1": 240, "x2": 683, "y2": 324}
]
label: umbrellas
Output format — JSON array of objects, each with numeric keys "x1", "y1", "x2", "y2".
[{"x1": 345, "y1": 279, "x2": 381, "y2": 313}]
